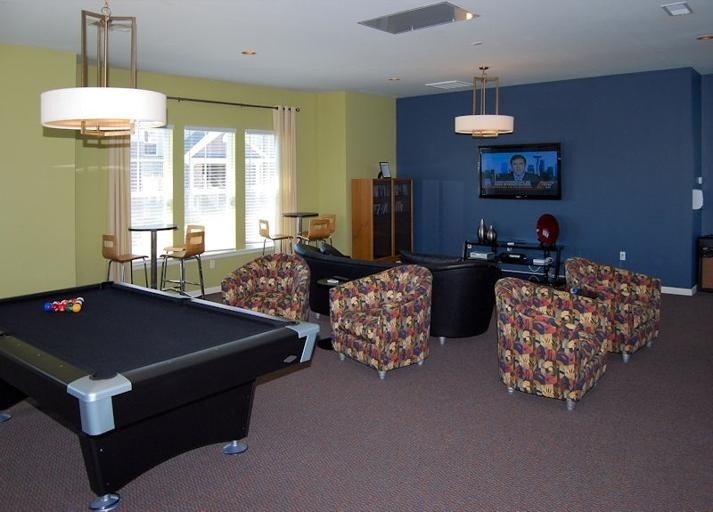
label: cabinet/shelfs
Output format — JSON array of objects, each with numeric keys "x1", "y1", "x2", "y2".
[
  {"x1": 352, "y1": 178, "x2": 413, "y2": 265},
  {"x1": 463, "y1": 239, "x2": 563, "y2": 281}
]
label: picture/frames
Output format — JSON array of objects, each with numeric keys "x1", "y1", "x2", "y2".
[{"x1": 377, "y1": 161, "x2": 390, "y2": 177}]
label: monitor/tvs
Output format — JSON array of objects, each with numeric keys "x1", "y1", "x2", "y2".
[{"x1": 477, "y1": 142, "x2": 562, "y2": 200}]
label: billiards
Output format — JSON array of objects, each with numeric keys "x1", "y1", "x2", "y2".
[{"x1": 44, "y1": 297, "x2": 84, "y2": 313}]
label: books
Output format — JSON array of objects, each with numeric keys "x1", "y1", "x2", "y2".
[{"x1": 374, "y1": 183, "x2": 409, "y2": 215}]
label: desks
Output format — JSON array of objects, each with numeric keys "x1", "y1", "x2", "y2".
[
  {"x1": 2, "y1": 281, "x2": 322, "y2": 512},
  {"x1": 281, "y1": 213, "x2": 320, "y2": 238}
]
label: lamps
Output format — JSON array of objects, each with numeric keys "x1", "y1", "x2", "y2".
[
  {"x1": 452, "y1": 66, "x2": 513, "y2": 138},
  {"x1": 38, "y1": 1, "x2": 167, "y2": 137}
]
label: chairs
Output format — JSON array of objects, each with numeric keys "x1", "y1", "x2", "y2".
[
  {"x1": 220, "y1": 252, "x2": 311, "y2": 319},
  {"x1": 102, "y1": 225, "x2": 205, "y2": 298},
  {"x1": 564, "y1": 257, "x2": 660, "y2": 361},
  {"x1": 295, "y1": 243, "x2": 396, "y2": 319},
  {"x1": 297, "y1": 220, "x2": 331, "y2": 248},
  {"x1": 258, "y1": 219, "x2": 293, "y2": 254},
  {"x1": 327, "y1": 263, "x2": 435, "y2": 380},
  {"x1": 319, "y1": 214, "x2": 336, "y2": 247},
  {"x1": 495, "y1": 277, "x2": 608, "y2": 411},
  {"x1": 398, "y1": 250, "x2": 500, "y2": 346}
]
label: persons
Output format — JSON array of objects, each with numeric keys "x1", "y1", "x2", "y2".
[{"x1": 496, "y1": 154, "x2": 546, "y2": 191}]
